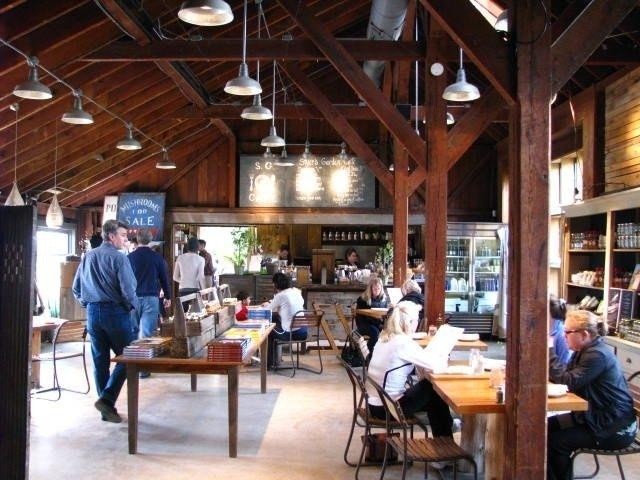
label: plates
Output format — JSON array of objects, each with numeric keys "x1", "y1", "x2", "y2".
[
  {"x1": 457, "y1": 333, "x2": 479, "y2": 343},
  {"x1": 412, "y1": 332, "x2": 427, "y2": 340},
  {"x1": 548, "y1": 384, "x2": 569, "y2": 397}
]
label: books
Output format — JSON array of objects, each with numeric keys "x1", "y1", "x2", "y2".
[
  {"x1": 122, "y1": 329, "x2": 252, "y2": 364},
  {"x1": 248, "y1": 309, "x2": 272, "y2": 314},
  {"x1": 234, "y1": 321, "x2": 262, "y2": 329},
  {"x1": 420, "y1": 321, "x2": 465, "y2": 361},
  {"x1": 248, "y1": 317, "x2": 271, "y2": 320},
  {"x1": 248, "y1": 314, "x2": 272, "y2": 317}
]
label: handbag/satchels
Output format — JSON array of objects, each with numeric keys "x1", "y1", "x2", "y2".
[
  {"x1": 359, "y1": 426, "x2": 402, "y2": 463},
  {"x1": 340, "y1": 334, "x2": 358, "y2": 363}
]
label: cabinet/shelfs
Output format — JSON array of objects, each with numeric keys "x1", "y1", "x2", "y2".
[
  {"x1": 560, "y1": 185, "x2": 640, "y2": 418},
  {"x1": 443, "y1": 230, "x2": 504, "y2": 336}
]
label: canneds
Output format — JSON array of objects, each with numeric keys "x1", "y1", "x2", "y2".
[{"x1": 617, "y1": 223, "x2": 640, "y2": 248}]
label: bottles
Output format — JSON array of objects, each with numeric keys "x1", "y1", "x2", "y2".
[
  {"x1": 476, "y1": 239, "x2": 500, "y2": 291},
  {"x1": 616, "y1": 317, "x2": 640, "y2": 344},
  {"x1": 615, "y1": 223, "x2": 639, "y2": 248},
  {"x1": 321, "y1": 229, "x2": 365, "y2": 240},
  {"x1": 495, "y1": 386, "x2": 503, "y2": 404},
  {"x1": 469, "y1": 349, "x2": 485, "y2": 374},
  {"x1": 569, "y1": 231, "x2": 598, "y2": 249},
  {"x1": 446, "y1": 239, "x2": 468, "y2": 291}
]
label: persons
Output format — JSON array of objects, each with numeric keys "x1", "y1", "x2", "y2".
[
  {"x1": 546, "y1": 310, "x2": 638, "y2": 480},
  {"x1": 197, "y1": 239, "x2": 217, "y2": 289},
  {"x1": 71, "y1": 220, "x2": 143, "y2": 424},
  {"x1": 122, "y1": 227, "x2": 175, "y2": 380},
  {"x1": 233, "y1": 288, "x2": 252, "y2": 320},
  {"x1": 364, "y1": 299, "x2": 455, "y2": 470},
  {"x1": 172, "y1": 237, "x2": 206, "y2": 313},
  {"x1": 395, "y1": 277, "x2": 425, "y2": 322},
  {"x1": 278, "y1": 243, "x2": 290, "y2": 258},
  {"x1": 261, "y1": 271, "x2": 309, "y2": 370},
  {"x1": 549, "y1": 297, "x2": 574, "y2": 371},
  {"x1": 338, "y1": 246, "x2": 362, "y2": 275},
  {"x1": 355, "y1": 277, "x2": 390, "y2": 366}
]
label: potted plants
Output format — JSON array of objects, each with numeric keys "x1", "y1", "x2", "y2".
[{"x1": 222, "y1": 225, "x2": 265, "y2": 276}]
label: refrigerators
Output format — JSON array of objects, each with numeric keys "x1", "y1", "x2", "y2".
[{"x1": 444, "y1": 221, "x2": 508, "y2": 333}]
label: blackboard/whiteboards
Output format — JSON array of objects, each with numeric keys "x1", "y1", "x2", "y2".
[{"x1": 116, "y1": 192, "x2": 166, "y2": 259}]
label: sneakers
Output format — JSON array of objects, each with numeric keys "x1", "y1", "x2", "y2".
[{"x1": 95, "y1": 397, "x2": 121, "y2": 423}]
